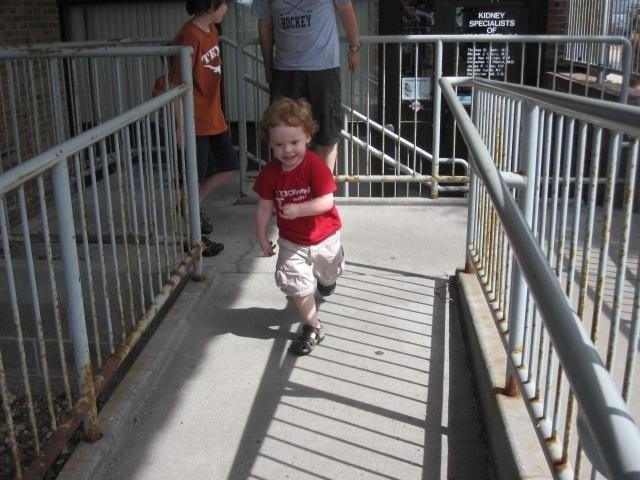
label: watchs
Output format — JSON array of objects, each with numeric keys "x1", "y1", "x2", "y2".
[{"x1": 348, "y1": 42, "x2": 361, "y2": 52}]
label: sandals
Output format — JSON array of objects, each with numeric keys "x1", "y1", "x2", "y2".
[
  {"x1": 317, "y1": 280, "x2": 336, "y2": 296},
  {"x1": 293, "y1": 319, "x2": 325, "y2": 355}
]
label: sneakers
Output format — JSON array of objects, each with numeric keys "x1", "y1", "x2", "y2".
[
  {"x1": 184, "y1": 235, "x2": 224, "y2": 256},
  {"x1": 176, "y1": 197, "x2": 213, "y2": 234}
]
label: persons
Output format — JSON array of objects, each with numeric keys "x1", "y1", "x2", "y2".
[
  {"x1": 250, "y1": 0, "x2": 361, "y2": 174},
  {"x1": 169, "y1": 0, "x2": 241, "y2": 257},
  {"x1": 252, "y1": 96, "x2": 345, "y2": 356}
]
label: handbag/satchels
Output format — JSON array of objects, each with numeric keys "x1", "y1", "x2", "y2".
[{"x1": 152, "y1": 74, "x2": 176, "y2": 127}]
